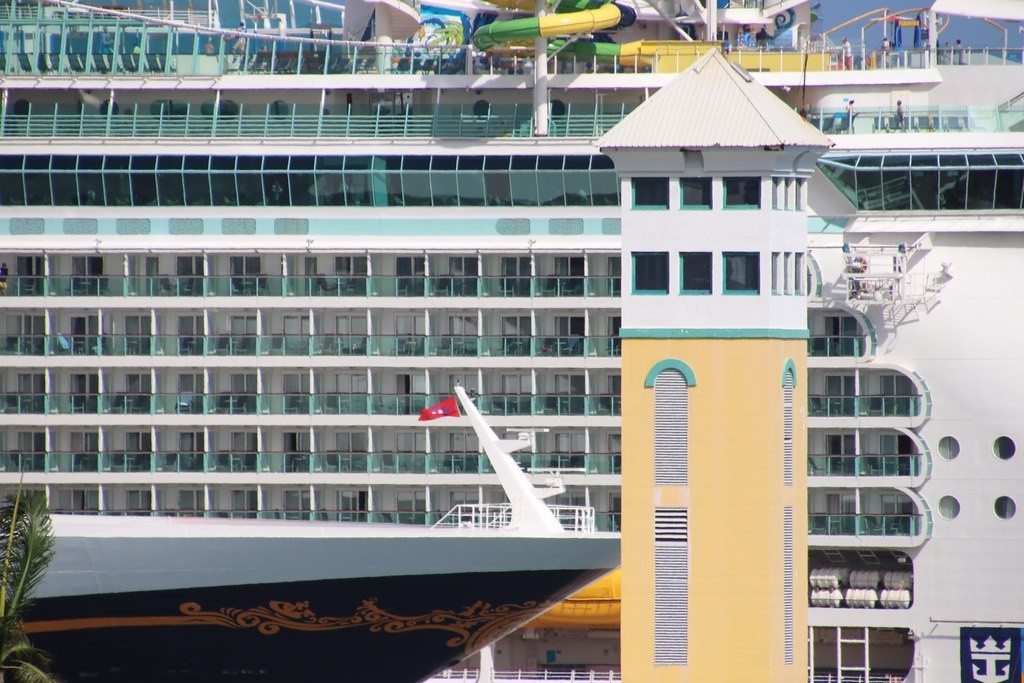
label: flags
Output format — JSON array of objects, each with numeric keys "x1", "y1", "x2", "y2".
[{"x1": 418, "y1": 396, "x2": 461, "y2": 421}]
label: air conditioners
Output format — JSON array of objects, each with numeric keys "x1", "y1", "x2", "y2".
[{"x1": 847, "y1": 251, "x2": 902, "y2": 273}]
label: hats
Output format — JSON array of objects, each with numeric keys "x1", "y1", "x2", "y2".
[{"x1": 842, "y1": 36, "x2": 847, "y2": 42}]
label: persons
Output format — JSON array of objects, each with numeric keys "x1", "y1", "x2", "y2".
[
  {"x1": 942, "y1": 39, "x2": 962, "y2": 65},
  {"x1": 846, "y1": 100, "x2": 857, "y2": 134},
  {"x1": 881, "y1": 35, "x2": 890, "y2": 68},
  {"x1": 841, "y1": 36, "x2": 852, "y2": 70},
  {"x1": 224, "y1": 21, "x2": 247, "y2": 54},
  {"x1": 101, "y1": 26, "x2": 114, "y2": 54},
  {"x1": 890, "y1": 100, "x2": 906, "y2": 133},
  {"x1": 201, "y1": 37, "x2": 214, "y2": 53},
  {"x1": 133, "y1": 43, "x2": 140, "y2": 53}
]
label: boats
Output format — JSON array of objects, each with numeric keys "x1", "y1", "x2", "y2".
[{"x1": 0, "y1": 386, "x2": 622, "y2": 683}]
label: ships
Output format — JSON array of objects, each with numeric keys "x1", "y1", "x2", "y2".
[{"x1": 0, "y1": 0, "x2": 1024, "y2": 683}]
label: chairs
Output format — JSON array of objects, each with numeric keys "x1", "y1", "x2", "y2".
[
  {"x1": 888, "y1": 514, "x2": 904, "y2": 535},
  {"x1": 811, "y1": 512, "x2": 828, "y2": 534},
  {"x1": 866, "y1": 454, "x2": 884, "y2": 475},
  {"x1": 812, "y1": 336, "x2": 828, "y2": 356},
  {"x1": 0, "y1": 336, "x2": 19, "y2": 356},
  {"x1": 867, "y1": 394, "x2": 887, "y2": 416},
  {"x1": 256, "y1": 51, "x2": 437, "y2": 76},
  {"x1": 25, "y1": 274, "x2": 38, "y2": 294},
  {"x1": 4, "y1": 392, "x2": 25, "y2": 412},
  {"x1": 862, "y1": 516, "x2": 884, "y2": 537},
  {"x1": 842, "y1": 512, "x2": 855, "y2": 533},
  {"x1": 56, "y1": 276, "x2": 621, "y2": 531},
  {"x1": 809, "y1": 457, "x2": 827, "y2": 476},
  {"x1": 810, "y1": 394, "x2": 827, "y2": 414},
  {"x1": 9, "y1": 449, "x2": 25, "y2": 472}
]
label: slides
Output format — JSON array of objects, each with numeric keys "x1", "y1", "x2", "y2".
[{"x1": 472, "y1": 0, "x2": 643, "y2": 66}]
